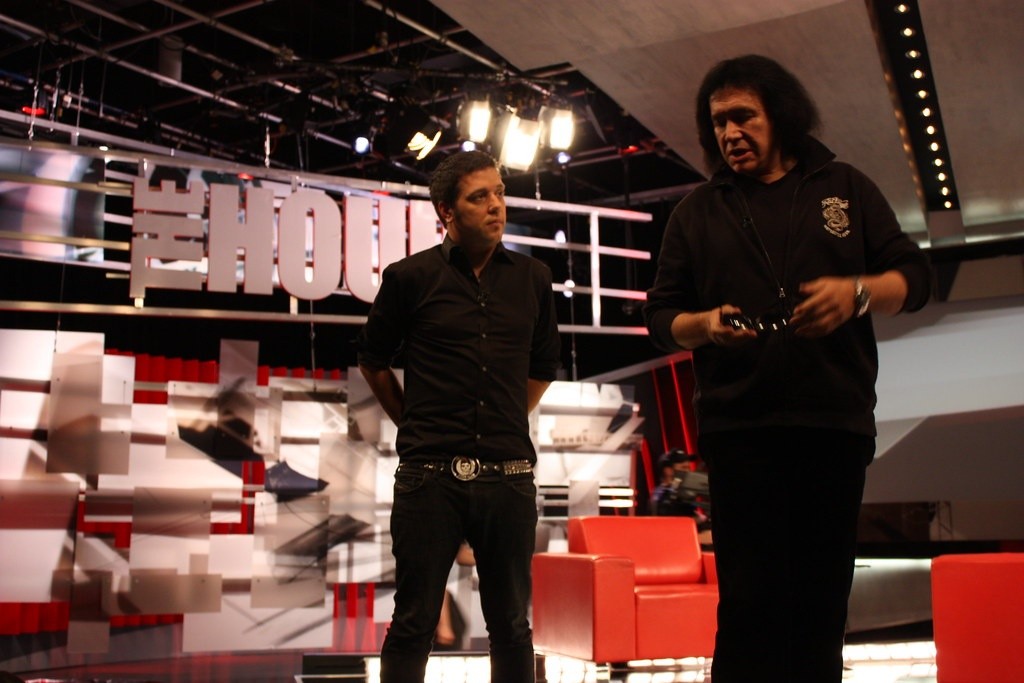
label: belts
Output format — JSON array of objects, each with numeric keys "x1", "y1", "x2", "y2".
[{"x1": 398, "y1": 456, "x2": 533, "y2": 482}]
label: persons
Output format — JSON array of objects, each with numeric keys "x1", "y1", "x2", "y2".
[
  {"x1": 642, "y1": 54, "x2": 934, "y2": 683},
  {"x1": 359, "y1": 152, "x2": 560, "y2": 683}
]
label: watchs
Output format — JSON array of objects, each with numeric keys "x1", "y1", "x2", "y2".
[{"x1": 850, "y1": 276, "x2": 870, "y2": 317}]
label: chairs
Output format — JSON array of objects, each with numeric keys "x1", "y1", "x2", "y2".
[
  {"x1": 530, "y1": 515, "x2": 720, "y2": 683},
  {"x1": 931, "y1": 553, "x2": 1024, "y2": 683}
]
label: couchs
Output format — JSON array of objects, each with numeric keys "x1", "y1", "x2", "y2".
[{"x1": 842, "y1": 557, "x2": 931, "y2": 634}]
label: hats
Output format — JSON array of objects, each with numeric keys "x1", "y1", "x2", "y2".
[{"x1": 659, "y1": 449, "x2": 698, "y2": 466}]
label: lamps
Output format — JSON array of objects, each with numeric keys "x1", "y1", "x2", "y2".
[{"x1": 386, "y1": 71, "x2": 577, "y2": 172}]
